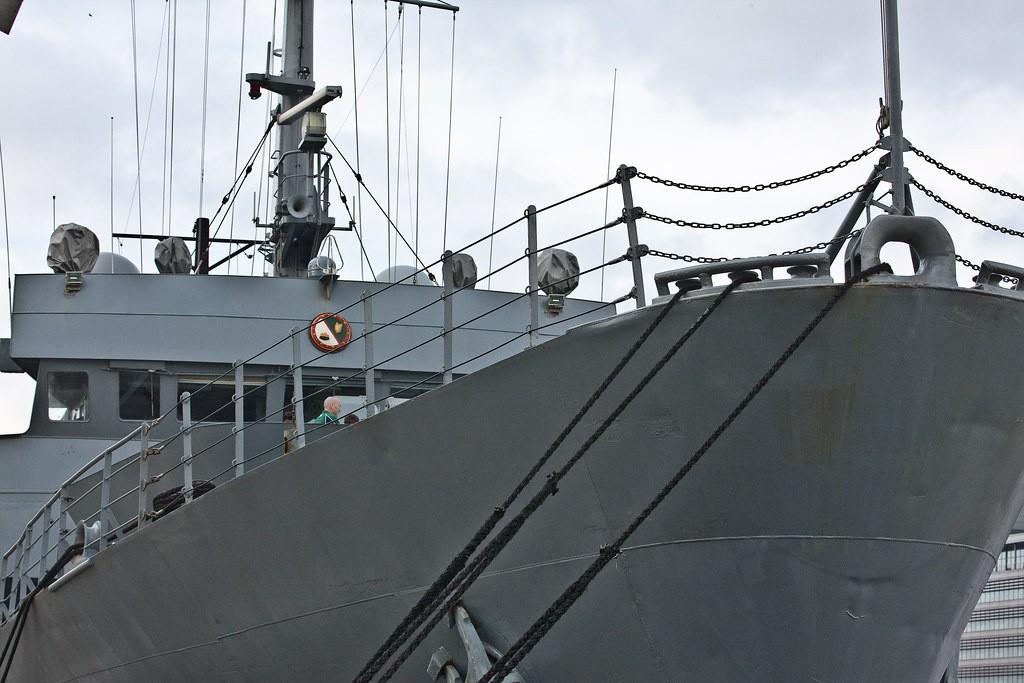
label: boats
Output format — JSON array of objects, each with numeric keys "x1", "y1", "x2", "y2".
[{"x1": 0, "y1": 0, "x2": 1024, "y2": 683}]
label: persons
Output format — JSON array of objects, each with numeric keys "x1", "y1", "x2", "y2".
[
  {"x1": 310, "y1": 397, "x2": 341, "y2": 424},
  {"x1": 343, "y1": 414, "x2": 359, "y2": 424}
]
